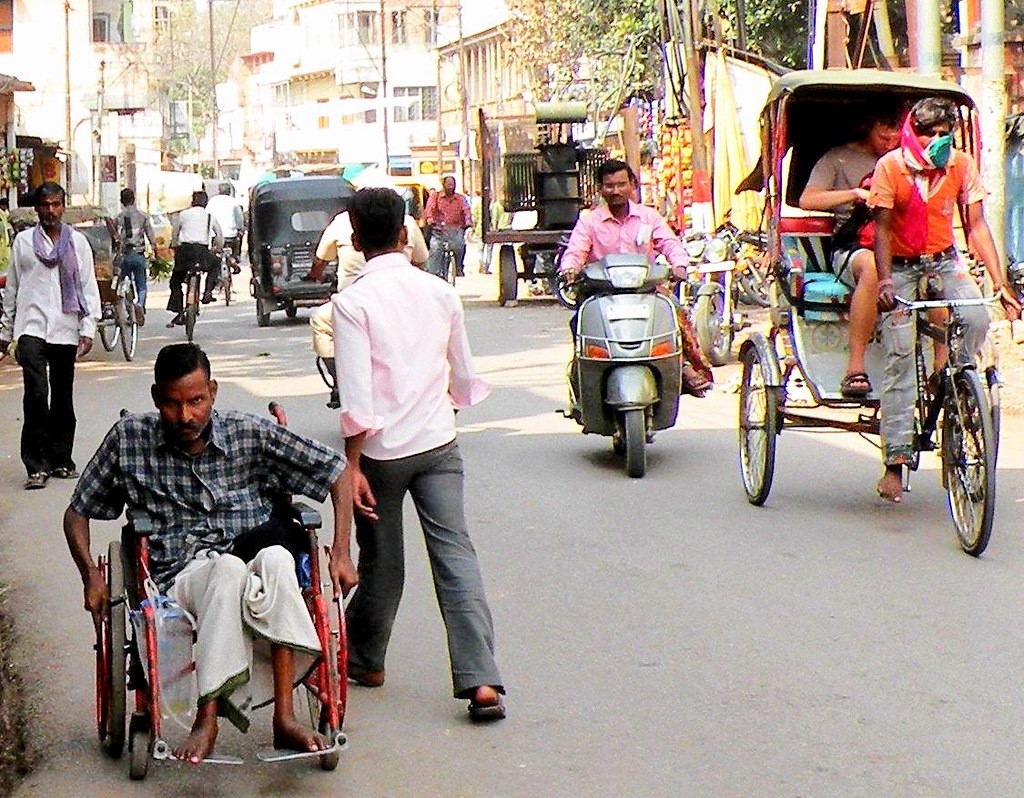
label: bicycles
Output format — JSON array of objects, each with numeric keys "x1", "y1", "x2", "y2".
[
  {"x1": 432, "y1": 224, "x2": 471, "y2": 287},
  {"x1": 169, "y1": 240, "x2": 235, "y2": 343}
]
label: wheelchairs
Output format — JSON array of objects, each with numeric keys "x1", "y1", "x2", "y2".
[{"x1": 93, "y1": 401, "x2": 350, "y2": 782}]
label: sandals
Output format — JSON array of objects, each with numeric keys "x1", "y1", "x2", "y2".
[
  {"x1": 682, "y1": 360, "x2": 710, "y2": 392},
  {"x1": 839, "y1": 371, "x2": 873, "y2": 396},
  {"x1": 467, "y1": 687, "x2": 505, "y2": 721},
  {"x1": 337, "y1": 650, "x2": 385, "y2": 686}
]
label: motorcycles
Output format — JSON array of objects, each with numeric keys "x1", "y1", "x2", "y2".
[
  {"x1": 244, "y1": 175, "x2": 358, "y2": 325},
  {"x1": 299, "y1": 270, "x2": 333, "y2": 389},
  {"x1": 551, "y1": 206, "x2": 773, "y2": 367}
]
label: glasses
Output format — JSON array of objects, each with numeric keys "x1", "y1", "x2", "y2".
[{"x1": 923, "y1": 128, "x2": 949, "y2": 137}]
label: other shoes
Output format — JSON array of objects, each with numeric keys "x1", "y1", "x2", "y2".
[
  {"x1": 173, "y1": 314, "x2": 185, "y2": 324},
  {"x1": 25, "y1": 467, "x2": 79, "y2": 489},
  {"x1": 135, "y1": 304, "x2": 145, "y2": 325},
  {"x1": 201, "y1": 294, "x2": 218, "y2": 305},
  {"x1": 330, "y1": 388, "x2": 339, "y2": 402},
  {"x1": 456, "y1": 270, "x2": 465, "y2": 277}
]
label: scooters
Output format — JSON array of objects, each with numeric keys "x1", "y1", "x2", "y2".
[{"x1": 555, "y1": 255, "x2": 695, "y2": 477}]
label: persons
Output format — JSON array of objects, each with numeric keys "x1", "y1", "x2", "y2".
[
  {"x1": 334, "y1": 188, "x2": 508, "y2": 722},
  {"x1": 801, "y1": 104, "x2": 967, "y2": 394},
  {"x1": 64, "y1": 345, "x2": 363, "y2": 764},
  {"x1": 867, "y1": 100, "x2": 1020, "y2": 504},
  {"x1": 0, "y1": 160, "x2": 710, "y2": 490}
]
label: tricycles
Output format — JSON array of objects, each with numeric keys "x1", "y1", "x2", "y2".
[
  {"x1": 736, "y1": 70, "x2": 1016, "y2": 556},
  {"x1": 12, "y1": 205, "x2": 138, "y2": 362}
]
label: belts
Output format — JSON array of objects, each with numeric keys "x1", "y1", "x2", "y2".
[{"x1": 890, "y1": 245, "x2": 955, "y2": 266}]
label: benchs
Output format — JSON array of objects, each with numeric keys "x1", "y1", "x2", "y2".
[{"x1": 778, "y1": 218, "x2": 852, "y2": 322}]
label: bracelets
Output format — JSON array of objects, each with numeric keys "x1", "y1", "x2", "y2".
[
  {"x1": 993, "y1": 283, "x2": 1005, "y2": 291},
  {"x1": 877, "y1": 278, "x2": 893, "y2": 290}
]
